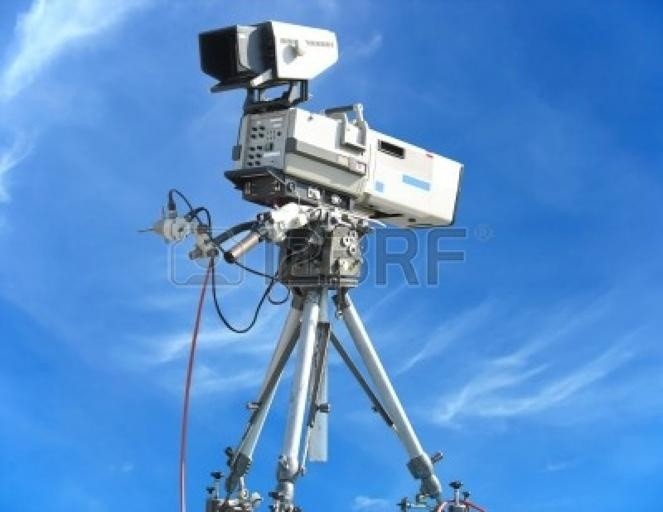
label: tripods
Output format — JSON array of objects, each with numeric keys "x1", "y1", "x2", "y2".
[{"x1": 204, "y1": 224, "x2": 479, "y2": 512}]
